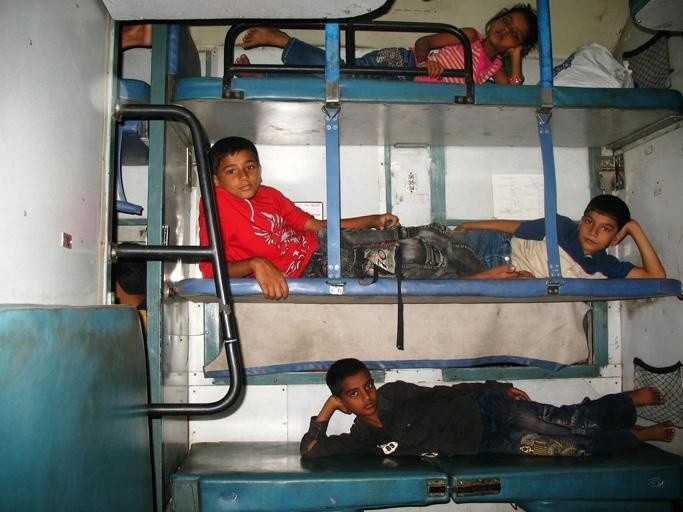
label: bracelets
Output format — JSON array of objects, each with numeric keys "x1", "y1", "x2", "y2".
[{"x1": 508, "y1": 72, "x2": 525, "y2": 85}]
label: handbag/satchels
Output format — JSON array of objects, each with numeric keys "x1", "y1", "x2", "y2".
[{"x1": 354, "y1": 241, "x2": 404, "y2": 351}]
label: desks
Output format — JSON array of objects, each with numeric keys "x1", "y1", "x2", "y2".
[{"x1": 1, "y1": 302, "x2": 160, "y2": 512}]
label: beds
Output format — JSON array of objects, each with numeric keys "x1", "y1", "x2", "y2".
[{"x1": 103, "y1": 18, "x2": 683, "y2": 511}]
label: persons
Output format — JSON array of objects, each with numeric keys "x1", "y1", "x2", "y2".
[
  {"x1": 198, "y1": 136, "x2": 535, "y2": 301},
  {"x1": 452, "y1": 194, "x2": 666, "y2": 279},
  {"x1": 234, "y1": 3, "x2": 537, "y2": 86},
  {"x1": 112, "y1": 242, "x2": 148, "y2": 328},
  {"x1": 299, "y1": 358, "x2": 676, "y2": 459}
]
